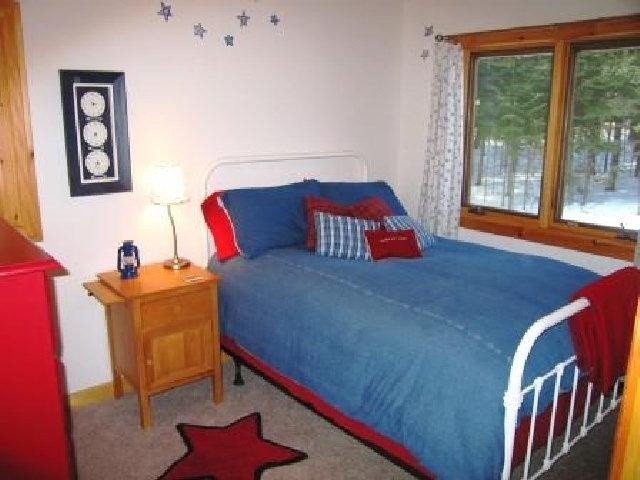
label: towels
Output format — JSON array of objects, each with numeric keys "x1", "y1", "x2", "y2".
[{"x1": 567, "y1": 265, "x2": 640, "y2": 398}]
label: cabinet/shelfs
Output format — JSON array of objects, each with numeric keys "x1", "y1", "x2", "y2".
[
  {"x1": 0, "y1": 0, "x2": 48, "y2": 244},
  {"x1": 0, "y1": 203, "x2": 84, "y2": 479}
]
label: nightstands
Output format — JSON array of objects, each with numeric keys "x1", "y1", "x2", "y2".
[{"x1": 81, "y1": 256, "x2": 224, "y2": 430}]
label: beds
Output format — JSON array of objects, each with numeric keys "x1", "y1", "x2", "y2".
[{"x1": 203, "y1": 151, "x2": 640, "y2": 479}]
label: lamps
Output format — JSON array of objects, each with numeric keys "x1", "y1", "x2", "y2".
[
  {"x1": 116, "y1": 238, "x2": 141, "y2": 281},
  {"x1": 148, "y1": 161, "x2": 195, "y2": 272}
]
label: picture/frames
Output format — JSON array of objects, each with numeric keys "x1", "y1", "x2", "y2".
[{"x1": 55, "y1": 67, "x2": 134, "y2": 199}]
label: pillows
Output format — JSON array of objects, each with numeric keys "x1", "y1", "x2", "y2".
[
  {"x1": 316, "y1": 179, "x2": 410, "y2": 220},
  {"x1": 303, "y1": 195, "x2": 397, "y2": 252},
  {"x1": 314, "y1": 210, "x2": 381, "y2": 263},
  {"x1": 216, "y1": 176, "x2": 332, "y2": 258},
  {"x1": 363, "y1": 228, "x2": 423, "y2": 259},
  {"x1": 199, "y1": 191, "x2": 239, "y2": 259},
  {"x1": 382, "y1": 212, "x2": 437, "y2": 249}
]
